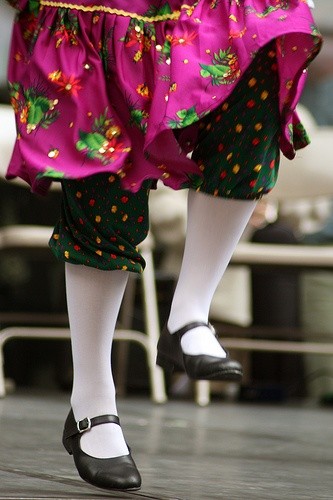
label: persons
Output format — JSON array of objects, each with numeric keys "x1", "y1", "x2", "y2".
[{"x1": 7, "y1": 0, "x2": 323, "y2": 492}]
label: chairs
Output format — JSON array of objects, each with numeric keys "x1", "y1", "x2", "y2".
[
  {"x1": 0, "y1": 104, "x2": 166, "y2": 402},
  {"x1": 173, "y1": 104, "x2": 329, "y2": 406}
]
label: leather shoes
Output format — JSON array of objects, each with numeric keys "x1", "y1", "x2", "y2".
[
  {"x1": 62, "y1": 407, "x2": 143, "y2": 493},
  {"x1": 156, "y1": 319, "x2": 244, "y2": 382}
]
